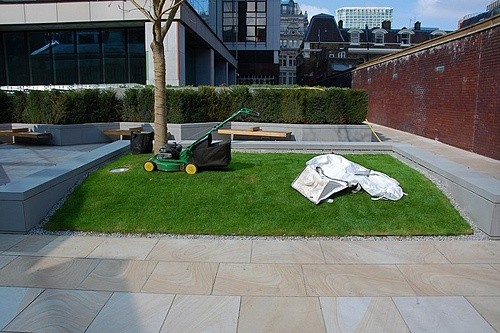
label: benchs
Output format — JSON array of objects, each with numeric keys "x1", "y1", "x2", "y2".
[
  {"x1": 0, "y1": 129, "x2": 51, "y2": 144},
  {"x1": 102, "y1": 128, "x2": 139, "y2": 141},
  {"x1": 217, "y1": 128, "x2": 294, "y2": 142}
]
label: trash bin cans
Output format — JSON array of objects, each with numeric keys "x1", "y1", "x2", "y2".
[{"x1": 129, "y1": 130, "x2": 155, "y2": 154}]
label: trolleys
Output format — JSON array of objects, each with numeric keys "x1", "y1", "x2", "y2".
[{"x1": 143, "y1": 107, "x2": 260, "y2": 174}]
